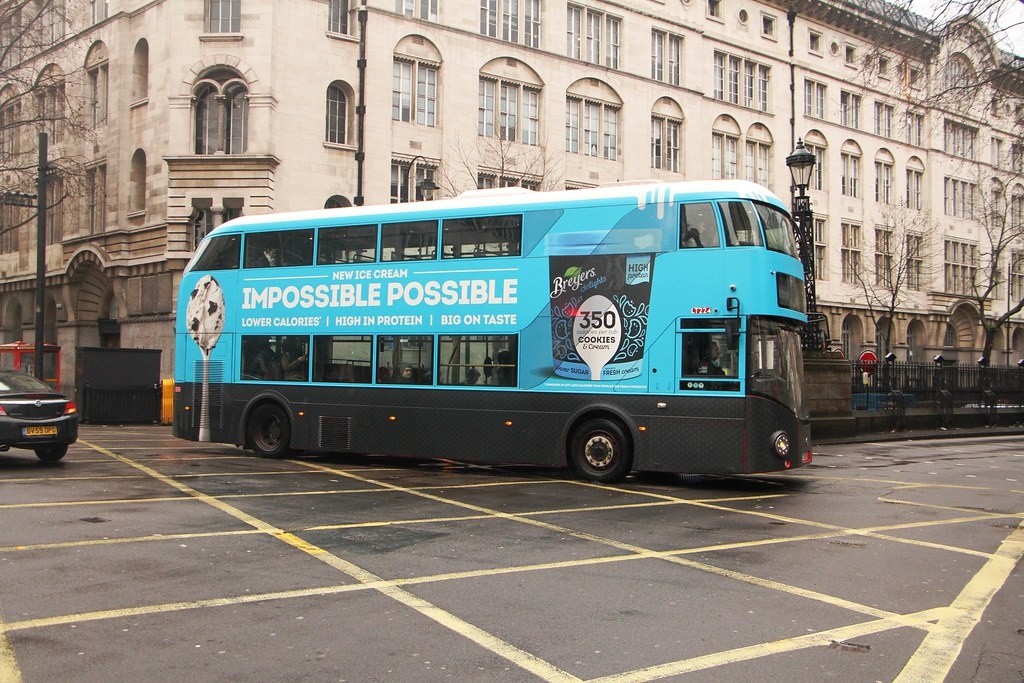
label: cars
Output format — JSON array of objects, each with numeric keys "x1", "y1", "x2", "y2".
[{"x1": 0, "y1": 367, "x2": 79, "y2": 462}]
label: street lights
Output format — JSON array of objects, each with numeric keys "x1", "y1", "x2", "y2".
[
  {"x1": 784, "y1": 136, "x2": 829, "y2": 352},
  {"x1": 401, "y1": 156, "x2": 440, "y2": 204}
]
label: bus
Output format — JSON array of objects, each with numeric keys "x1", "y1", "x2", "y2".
[{"x1": 171, "y1": 179, "x2": 814, "y2": 485}]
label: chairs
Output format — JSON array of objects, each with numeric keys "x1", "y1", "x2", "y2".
[{"x1": 320, "y1": 247, "x2": 495, "y2": 262}]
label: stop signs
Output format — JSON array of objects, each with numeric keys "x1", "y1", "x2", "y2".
[{"x1": 858, "y1": 350, "x2": 878, "y2": 374}]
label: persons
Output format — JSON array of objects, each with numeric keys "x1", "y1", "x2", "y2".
[
  {"x1": 248, "y1": 336, "x2": 481, "y2": 385},
  {"x1": 256, "y1": 245, "x2": 276, "y2": 267},
  {"x1": 696, "y1": 341, "x2": 728, "y2": 376}
]
